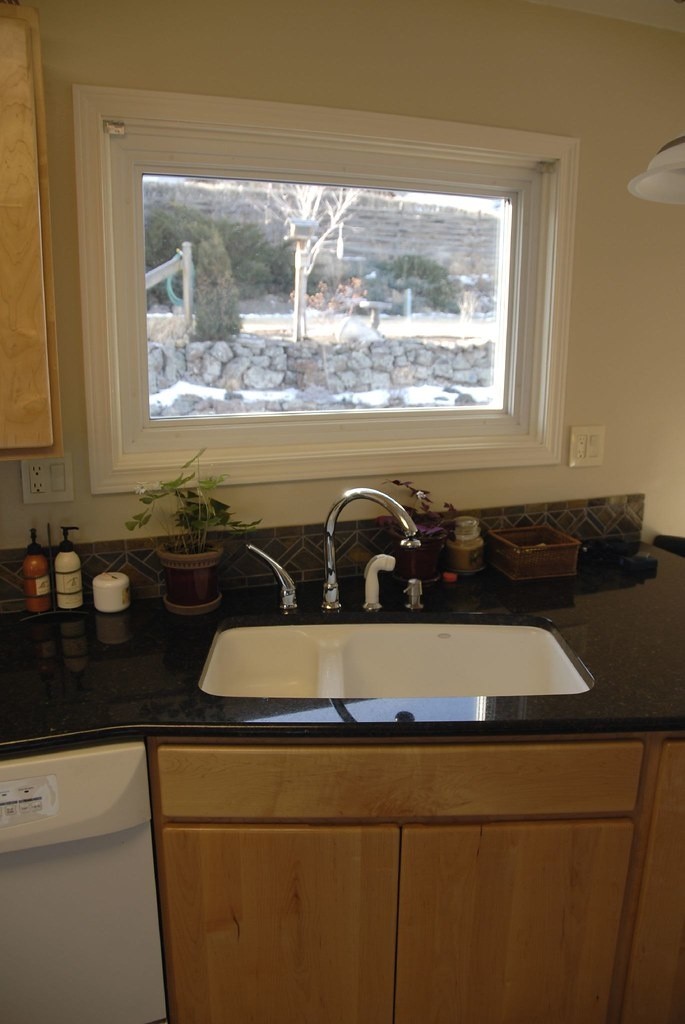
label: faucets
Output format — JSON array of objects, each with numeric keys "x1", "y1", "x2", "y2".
[{"x1": 319, "y1": 485, "x2": 421, "y2": 613}]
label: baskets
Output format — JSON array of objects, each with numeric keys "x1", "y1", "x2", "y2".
[{"x1": 487, "y1": 525, "x2": 583, "y2": 580}]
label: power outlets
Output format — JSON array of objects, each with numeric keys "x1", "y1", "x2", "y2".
[
  {"x1": 568, "y1": 425, "x2": 605, "y2": 468},
  {"x1": 20, "y1": 452, "x2": 74, "y2": 504}
]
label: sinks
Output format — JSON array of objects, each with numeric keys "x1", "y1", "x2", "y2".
[{"x1": 196, "y1": 604, "x2": 596, "y2": 702}]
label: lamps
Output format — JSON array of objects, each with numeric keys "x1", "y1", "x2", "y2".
[{"x1": 627, "y1": 135, "x2": 685, "y2": 204}]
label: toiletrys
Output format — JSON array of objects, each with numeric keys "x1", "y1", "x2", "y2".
[{"x1": 21, "y1": 525, "x2": 85, "y2": 614}]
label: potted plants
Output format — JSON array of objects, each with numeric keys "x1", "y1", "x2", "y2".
[
  {"x1": 377, "y1": 478, "x2": 458, "y2": 583},
  {"x1": 124, "y1": 445, "x2": 262, "y2": 616}
]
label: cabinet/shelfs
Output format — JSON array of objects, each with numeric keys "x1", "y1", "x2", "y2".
[
  {"x1": 148, "y1": 732, "x2": 685, "y2": 1024},
  {"x1": 0, "y1": 2, "x2": 62, "y2": 459}
]
